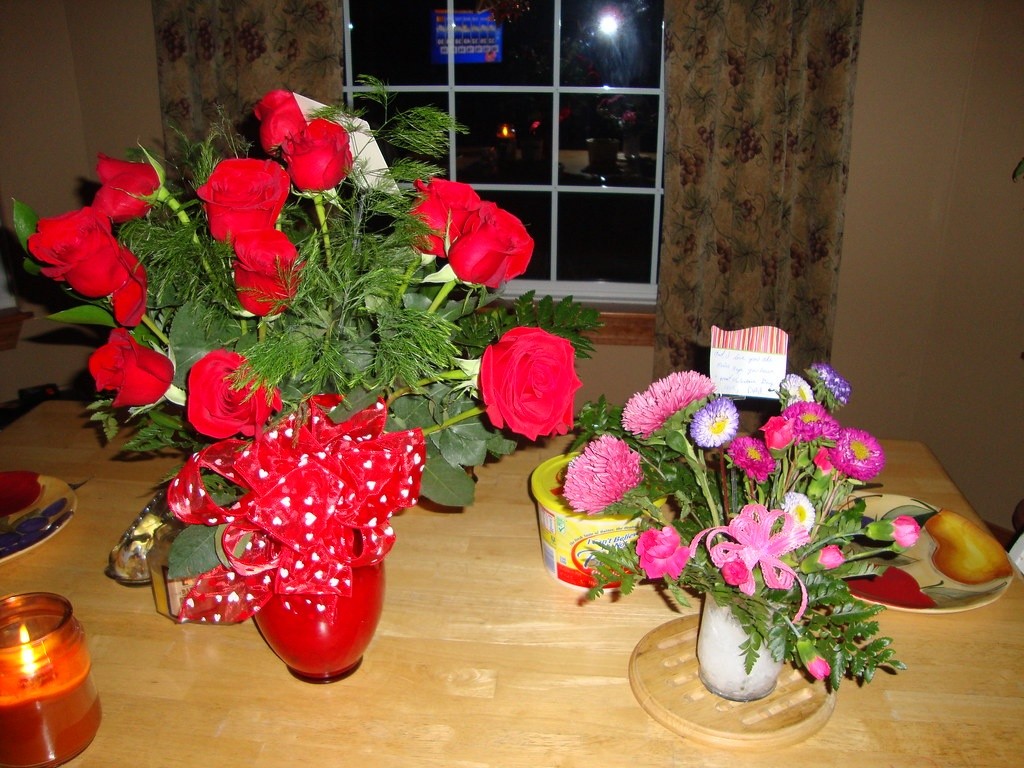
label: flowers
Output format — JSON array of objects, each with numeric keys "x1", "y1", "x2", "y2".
[
  {"x1": 9, "y1": 75, "x2": 586, "y2": 579},
  {"x1": 560, "y1": 356, "x2": 924, "y2": 694}
]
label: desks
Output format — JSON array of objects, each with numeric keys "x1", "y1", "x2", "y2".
[{"x1": 0, "y1": 387, "x2": 1024, "y2": 768}]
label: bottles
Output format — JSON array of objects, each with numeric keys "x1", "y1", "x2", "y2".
[{"x1": 0, "y1": 587, "x2": 102, "y2": 768}]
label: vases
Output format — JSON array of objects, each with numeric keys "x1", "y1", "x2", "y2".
[
  {"x1": 693, "y1": 581, "x2": 785, "y2": 702},
  {"x1": 248, "y1": 526, "x2": 390, "y2": 684}
]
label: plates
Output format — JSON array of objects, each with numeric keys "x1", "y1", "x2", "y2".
[
  {"x1": 843, "y1": 491, "x2": 1015, "y2": 614},
  {"x1": 0, "y1": 468, "x2": 78, "y2": 567}
]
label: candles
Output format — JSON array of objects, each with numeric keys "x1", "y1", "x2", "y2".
[{"x1": 0, "y1": 592, "x2": 103, "y2": 768}]
